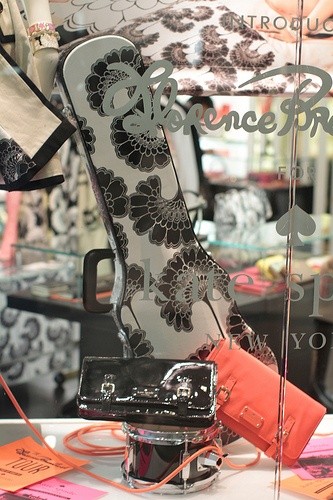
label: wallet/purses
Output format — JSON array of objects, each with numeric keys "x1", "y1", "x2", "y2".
[
  {"x1": 204, "y1": 339, "x2": 326, "y2": 467},
  {"x1": 76, "y1": 357, "x2": 218, "y2": 429}
]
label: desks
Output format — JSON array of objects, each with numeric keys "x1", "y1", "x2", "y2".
[{"x1": 0, "y1": 413, "x2": 333, "y2": 499}]
